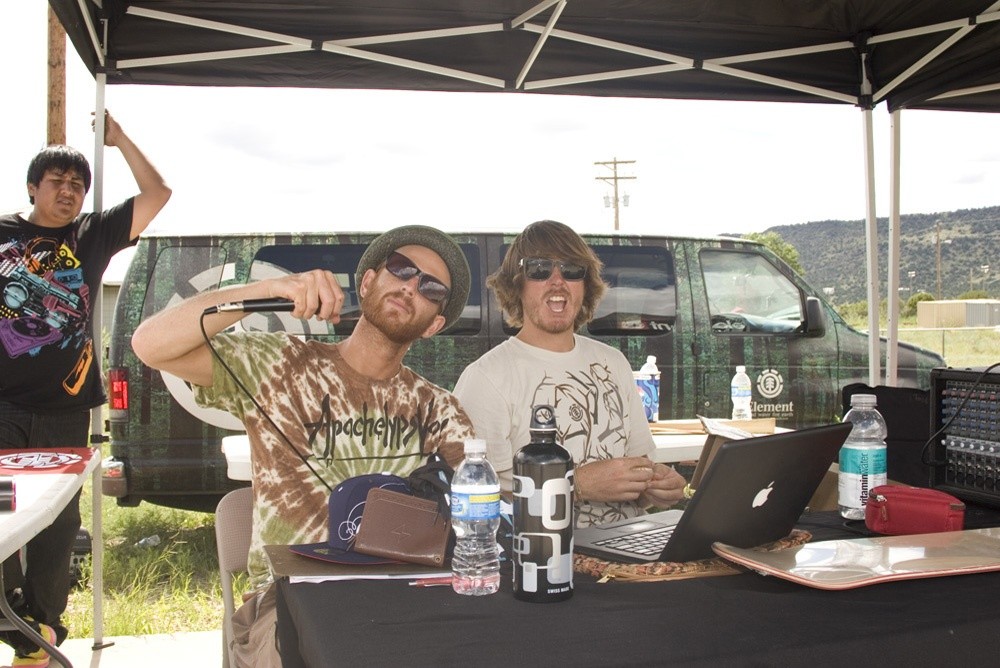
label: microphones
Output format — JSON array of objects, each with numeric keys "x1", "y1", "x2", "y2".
[{"x1": 204, "y1": 295, "x2": 322, "y2": 316}]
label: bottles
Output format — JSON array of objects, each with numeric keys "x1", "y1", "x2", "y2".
[
  {"x1": 449, "y1": 438, "x2": 502, "y2": 596},
  {"x1": 640, "y1": 355, "x2": 659, "y2": 372},
  {"x1": 510, "y1": 404, "x2": 575, "y2": 603},
  {"x1": 730, "y1": 365, "x2": 753, "y2": 421},
  {"x1": 839, "y1": 393, "x2": 889, "y2": 520}
]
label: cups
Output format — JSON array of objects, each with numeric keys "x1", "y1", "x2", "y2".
[{"x1": 631, "y1": 370, "x2": 661, "y2": 425}]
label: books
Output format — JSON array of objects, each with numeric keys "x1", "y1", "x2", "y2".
[{"x1": 289, "y1": 574, "x2": 459, "y2": 585}]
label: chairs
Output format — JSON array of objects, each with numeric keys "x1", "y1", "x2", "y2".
[
  {"x1": 841, "y1": 383, "x2": 929, "y2": 490},
  {"x1": 216, "y1": 487, "x2": 256, "y2": 668}
]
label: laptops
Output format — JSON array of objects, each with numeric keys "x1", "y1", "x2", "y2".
[{"x1": 571, "y1": 420, "x2": 854, "y2": 566}]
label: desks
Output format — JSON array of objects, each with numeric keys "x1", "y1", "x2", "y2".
[
  {"x1": 276, "y1": 510, "x2": 1000, "y2": 668},
  {"x1": 0, "y1": 447, "x2": 101, "y2": 668},
  {"x1": 224, "y1": 419, "x2": 796, "y2": 481}
]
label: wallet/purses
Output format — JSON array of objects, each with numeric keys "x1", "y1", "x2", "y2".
[{"x1": 862, "y1": 485, "x2": 966, "y2": 535}]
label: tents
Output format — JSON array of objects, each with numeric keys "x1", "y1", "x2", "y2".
[{"x1": 51, "y1": 0, "x2": 1000, "y2": 668}]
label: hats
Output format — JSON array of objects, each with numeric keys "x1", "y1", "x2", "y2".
[
  {"x1": 288, "y1": 471, "x2": 442, "y2": 566},
  {"x1": 354, "y1": 224, "x2": 471, "y2": 334}
]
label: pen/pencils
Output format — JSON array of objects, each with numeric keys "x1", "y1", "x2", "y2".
[{"x1": 409, "y1": 578, "x2": 453, "y2": 587}]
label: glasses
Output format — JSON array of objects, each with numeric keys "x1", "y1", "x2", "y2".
[
  {"x1": 517, "y1": 255, "x2": 588, "y2": 282},
  {"x1": 380, "y1": 250, "x2": 450, "y2": 303}
]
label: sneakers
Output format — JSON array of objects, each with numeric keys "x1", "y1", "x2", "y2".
[{"x1": 11, "y1": 624, "x2": 58, "y2": 668}]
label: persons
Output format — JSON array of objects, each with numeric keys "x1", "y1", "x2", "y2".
[
  {"x1": 0, "y1": 108, "x2": 151, "y2": 668},
  {"x1": 449, "y1": 219, "x2": 686, "y2": 527},
  {"x1": 132, "y1": 226, "x2": 480, "y2": 668}
]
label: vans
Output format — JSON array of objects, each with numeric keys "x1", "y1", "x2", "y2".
[{"x1": 89, "y1": 223, "x2": 950, "y2": 519}]
